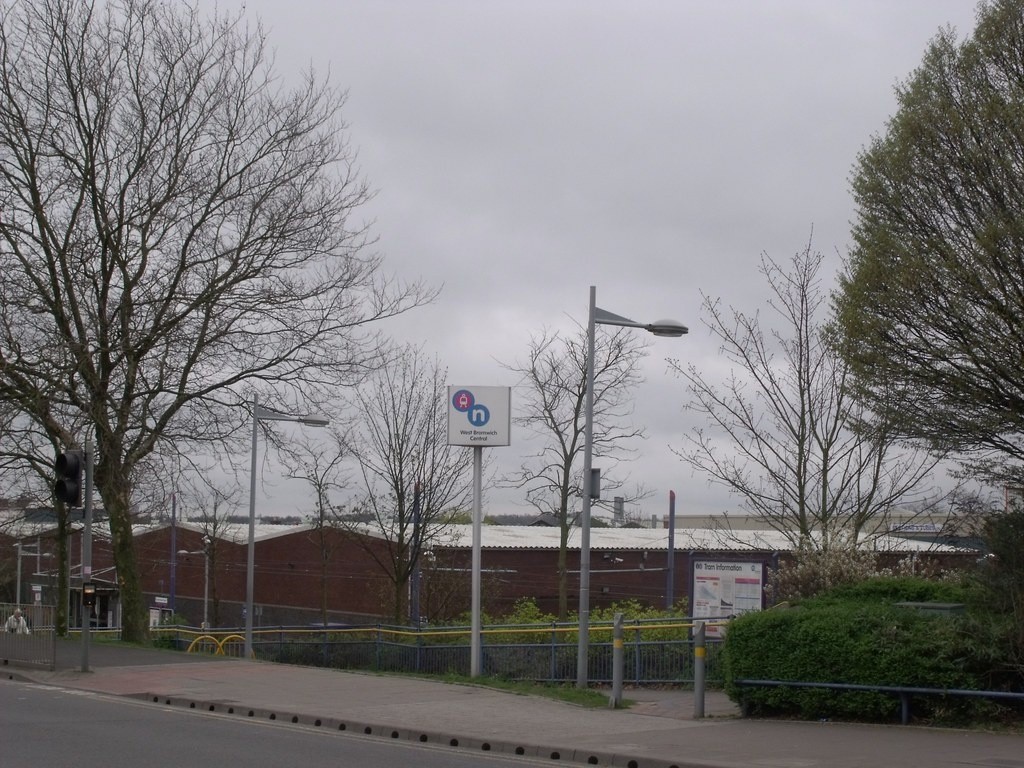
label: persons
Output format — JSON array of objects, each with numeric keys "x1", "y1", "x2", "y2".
[{"x1": 4, "y1": 608, "x2": 30, "y2": 634}]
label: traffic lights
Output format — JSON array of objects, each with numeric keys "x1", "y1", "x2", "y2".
[{"x1": 54, "y1": 452, "x2": 81, "y2": 510}]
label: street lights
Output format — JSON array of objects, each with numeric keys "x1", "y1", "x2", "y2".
[
  {"x1": 244, "y1": 406, "x2": 331, "y2": 660},
  {"x1": 176, "y1": 535, "x2": 210, "y2": 629},
  {"x1": 576, "y1": 307, "x2": 689, "y2": 695},
  {"x1": 16, "y1": 542, "x2": 56, "y2": 607},
  {"x1": 12, "y1": 537, "x2": 42, "y2": 574}
]
